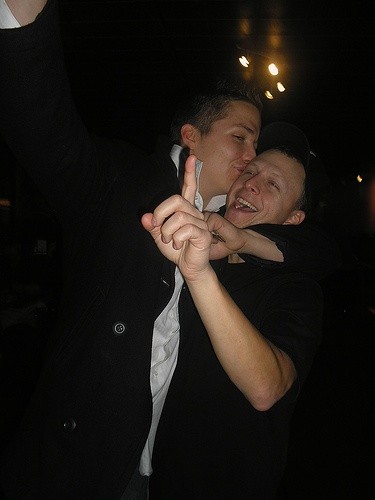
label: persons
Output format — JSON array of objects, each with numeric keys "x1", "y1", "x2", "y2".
[
  {"x1": 39, "y1": 84, "x2": 322, "y2": 365},
  {"x1": 40, "y1": 145, "x2": 321, "y2": 499}
]
